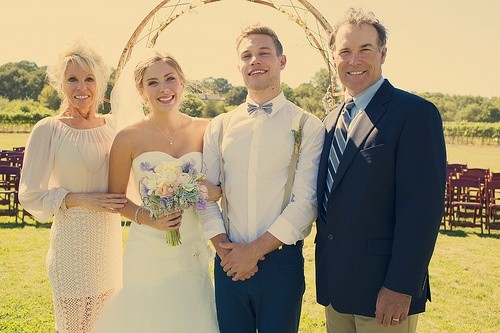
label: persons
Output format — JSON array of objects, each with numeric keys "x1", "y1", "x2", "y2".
[
  {"x1": 196, "y1": 26, "x2": 326, "y2": 333},
  {"x1": 315, "y1": 17, "x2": 446, "y2": 333},
  {"x1": 19, "y1": 44, "x2": 128, "y2": 333},
  {"x1": 93, "y1": 52, "x2": 220, "y2": 333}
]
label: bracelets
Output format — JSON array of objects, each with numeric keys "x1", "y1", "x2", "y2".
[
  {"x1": 134, "y1": 204, "x2": 145, "y2": 225},
  {"x1": 61, "y1": 193, "x2": 70, "y2": 211}
]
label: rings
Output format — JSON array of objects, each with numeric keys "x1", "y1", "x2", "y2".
[
  {"x1": 392, "y1": 317, "x2": 400, "y2": 322},
  {"x1": 227, "y1": 269, "x2": 234, "y2": 276},
  {"x1": 206, "y1": 193, "x2": 210, "y2": 200}
]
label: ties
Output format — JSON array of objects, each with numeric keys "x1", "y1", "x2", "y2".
[{"x1": 322, "y1": 99, "x2": 354, "y2": 221}]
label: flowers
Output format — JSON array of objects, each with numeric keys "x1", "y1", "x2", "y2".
[{"x1": 139, "y1": 160, "x2": 208, "y2": 246}]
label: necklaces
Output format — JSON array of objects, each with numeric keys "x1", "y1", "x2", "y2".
[{"x1": 146, "y1": 115, "x2": 188, "y2": 146}]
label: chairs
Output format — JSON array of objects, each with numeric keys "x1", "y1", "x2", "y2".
[
  {"x1": 440, "y1": 162, "x2": 500, "y2": 235},
  {"x1": 0, "y1": 147, "x2": 26, "y2": 226}
]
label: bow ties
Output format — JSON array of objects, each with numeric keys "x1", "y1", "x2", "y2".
[{"x1": 246, "y1": 102, "x2": 273, "y2": 116}]
